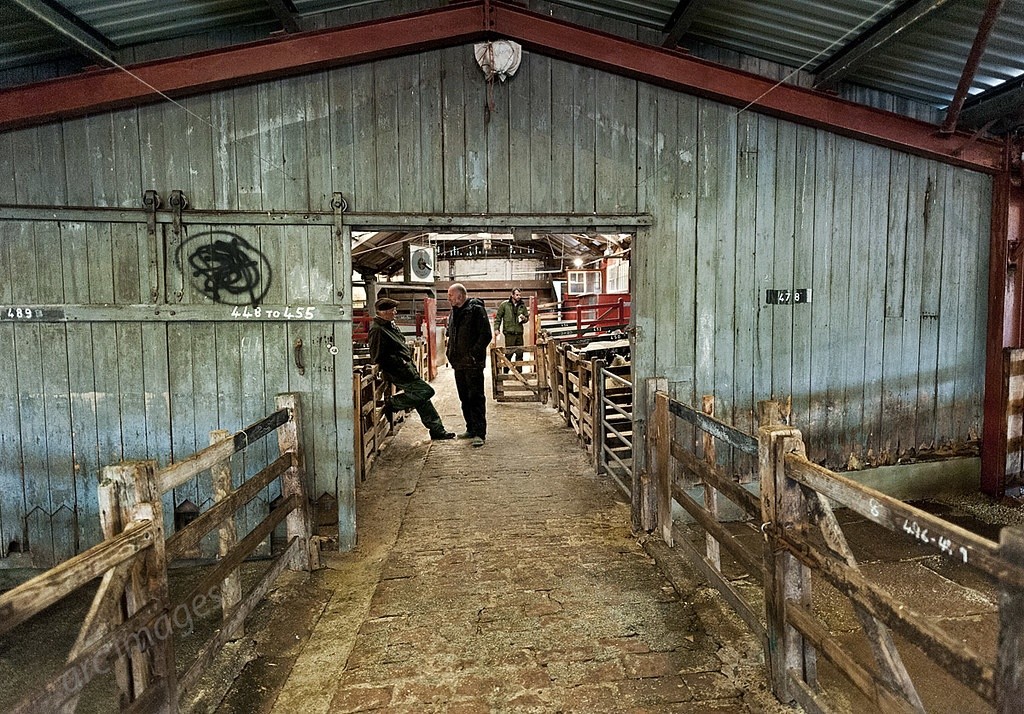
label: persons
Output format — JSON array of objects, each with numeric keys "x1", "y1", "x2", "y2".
[
  {"x1": 368, "y1": 298, "x2": 456, "y2": 440},
  {"x1": 493, "y1": 287, "x2": 529, "y2": 375},
  {"x1": 444, "y1": 283, "x2": 492, "y2": 446}
]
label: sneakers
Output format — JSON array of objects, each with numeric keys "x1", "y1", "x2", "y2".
[
  {"x1": 432, "y1": 432, "x2": 455, "y2": 439},
  {"x1": 384, "y1": 395, "x2": 393, "y2": 422},
  {"x1": 457, "y1": 432, "x2": 475, "y2": 438},
  {"x1": 472, "y1": 437, "x2": 486, "y2": 445}
]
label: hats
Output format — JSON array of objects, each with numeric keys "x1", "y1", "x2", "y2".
[{"x1": 374, "y1": 298, "x2": 400, "y2": 311}]
